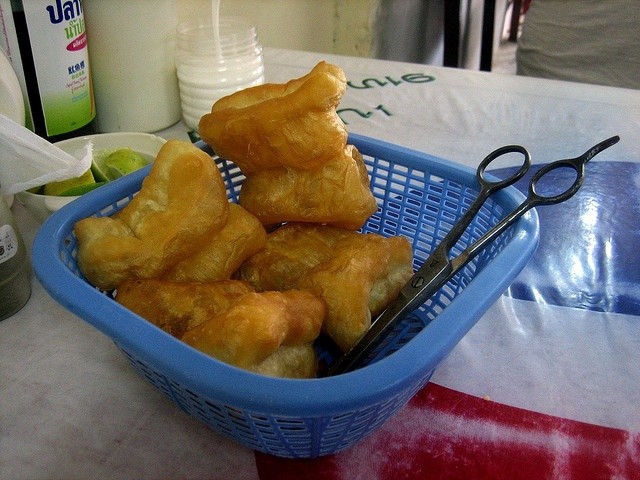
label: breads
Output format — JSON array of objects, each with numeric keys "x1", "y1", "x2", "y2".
[
  {"x1": 116, "y1": 277, "x2": 253, "y2": 340},
  {"x1": 182, "y1": 289, "x2": 324, "y2": 379},
  {"x1": 239, "y1": 221, "x2": 414, "y2": 350},
  {"x1": 197, "y1": 58, "x2": 350, "y2": 178},
  {"x1": 164, "y1": 201, "x2": 266, "y2": 279},
  {"x1": 239, "y1": 143, "x2": 377, "y2": 232},
  {"x1": 73, "y1": 140, "x2": 231, "y2": 293}
]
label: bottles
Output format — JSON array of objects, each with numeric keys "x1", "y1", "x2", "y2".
[
  {"x1": 84, "y1": 0, "x2": 182, "y2": 134},
  {"x1": 0, "y1": 192, "x2": 31, "y2": 322},
  {"x1": 0, "y1": 1, "x2": 96, "y2": 144}
]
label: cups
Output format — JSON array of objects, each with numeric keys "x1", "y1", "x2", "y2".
[{"x1": 175, "y1": 16, "x2": 265, "y2": 129}]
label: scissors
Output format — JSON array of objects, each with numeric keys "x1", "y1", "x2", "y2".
[{"x1": 329, "y1": 135, "x2": 620, "y2": 371}]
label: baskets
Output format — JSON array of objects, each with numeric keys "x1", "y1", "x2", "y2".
[{"x1": 32, "y1": 132, "x2": 540, "y2": 459}]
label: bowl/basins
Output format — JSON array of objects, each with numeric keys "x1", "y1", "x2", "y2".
[{"x1": 16, "y1": 131, "x2": 168, "y2": 224}]
